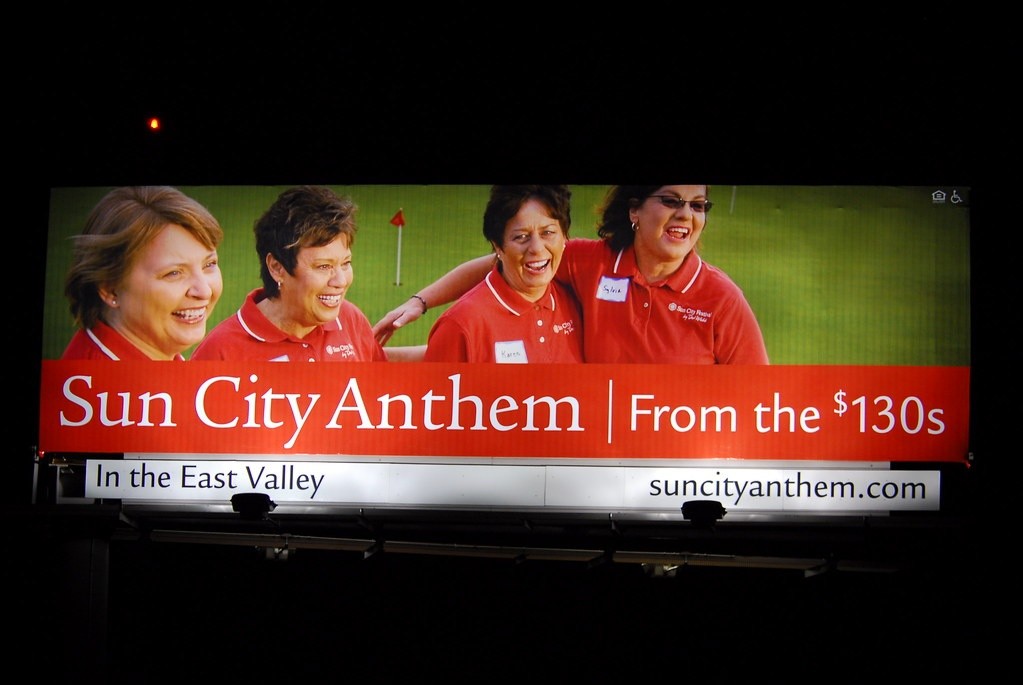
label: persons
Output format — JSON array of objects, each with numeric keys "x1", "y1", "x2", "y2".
[
  {"x1": 189, "y1": 186, "x2": 429, "y2": 363},
  {"x1": 57, "y1": 186, "x2": 223, "y2": 363},
  {"x1": 370, "y1": 185, "x2": 770, "y2": 363},
  {"x1": 422, "y1": 185, "x2": 584, "y2": 363}
]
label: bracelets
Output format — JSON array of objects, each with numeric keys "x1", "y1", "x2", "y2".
[{"x1": 411, "y1": 295, "x2": 427, "y2": 314}]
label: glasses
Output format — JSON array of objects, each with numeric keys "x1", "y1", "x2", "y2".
[{"x1": 649, "y1": 195, "x2": 714, "y2": 212}]
